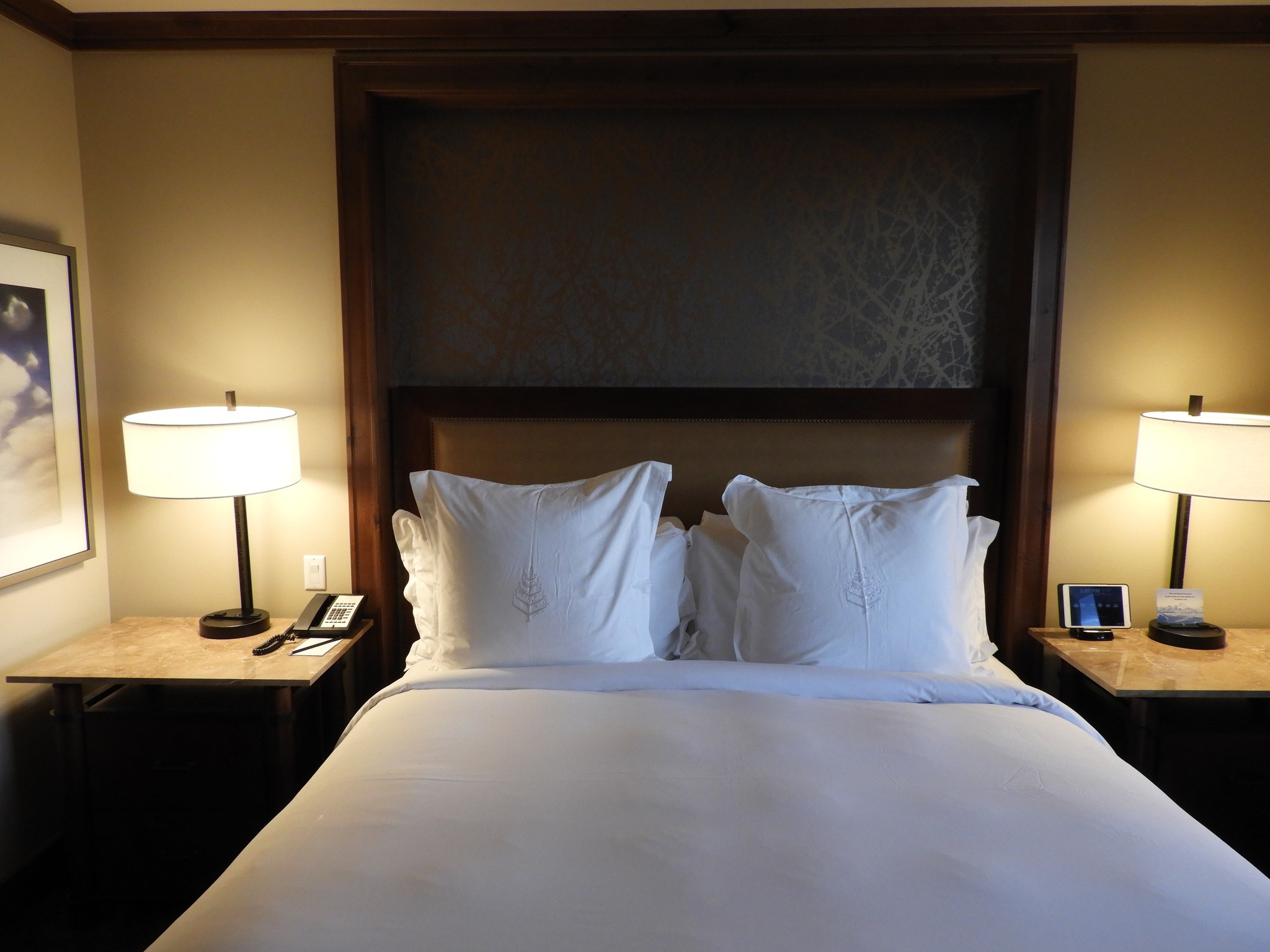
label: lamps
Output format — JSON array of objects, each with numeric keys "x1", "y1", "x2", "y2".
[
  {"x1": 121, "y1": 388, "x2": 303, "y2": 641},
  {"x1": 1125, "y1": 392, "x2": 1270, "y2": 652}
]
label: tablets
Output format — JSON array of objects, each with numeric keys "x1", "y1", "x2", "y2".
[{"x1": 1058, "y1": 583, "x2": 1132, "y2": 629}]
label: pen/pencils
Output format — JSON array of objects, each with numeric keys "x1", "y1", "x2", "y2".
[{"x1": 289, "y1": 636, "x2": 342, "y2": 654}]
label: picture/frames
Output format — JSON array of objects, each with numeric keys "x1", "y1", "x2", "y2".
[{"x1": 0, "y1": 227, "x2": 99, "y2": 597}]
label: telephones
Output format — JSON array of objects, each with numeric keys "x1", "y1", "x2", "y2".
[{"x1": 292, "y1": 593, "x2": 368, "y2": 636}]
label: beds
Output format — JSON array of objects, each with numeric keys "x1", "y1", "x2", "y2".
[{"x1": 134, "y1": 384, "x2": 1270, "y2": 952}]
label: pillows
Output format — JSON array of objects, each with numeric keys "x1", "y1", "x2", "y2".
[
  {"x1": 391, "y1": 508, "x2": 693, "y2": 674},
  {"x1": 717, "y1": 472, "x2": 981, "y2": 667},
  {"x1": 683, "y1": 508, "x2": 1006, "y2": 665},
  {"x1": 403, "y1": 459, "x2": 675, "y2": 668}
]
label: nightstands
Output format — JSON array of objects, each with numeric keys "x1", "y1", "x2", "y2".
[
  {"x1": 5, "y1": 614, "x2": 376, "y2": 803},
  {"x1": 1024, "y1": 624, "x2": 1270, "y2": 778}
]
